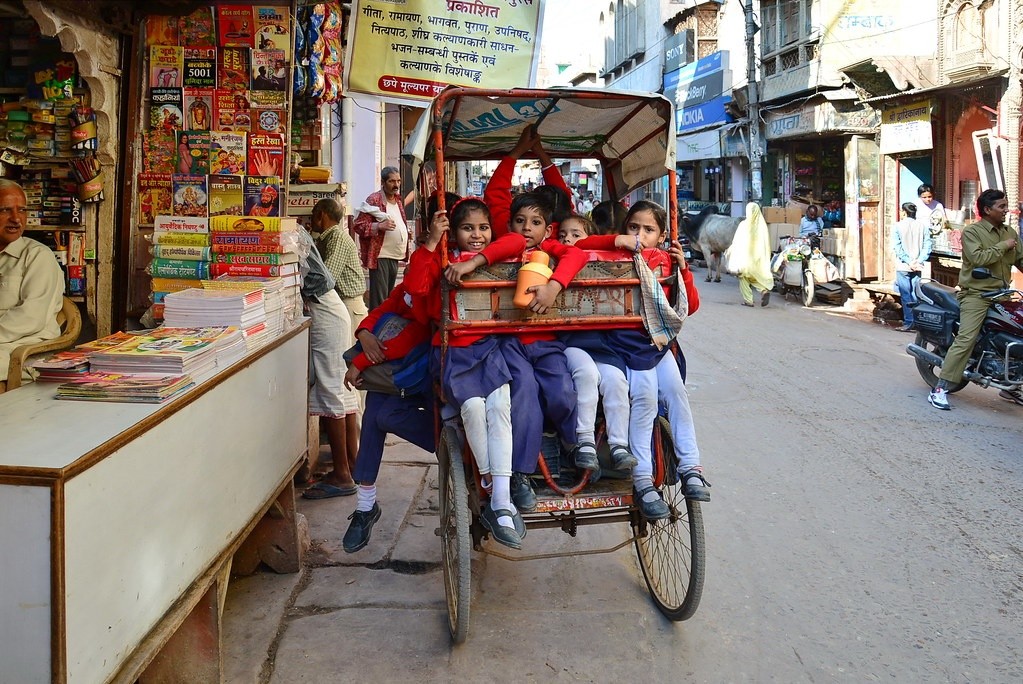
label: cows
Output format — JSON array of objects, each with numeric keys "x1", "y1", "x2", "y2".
[{"x1": 678, "y1": 204, "x2": 746, "y2": 283}]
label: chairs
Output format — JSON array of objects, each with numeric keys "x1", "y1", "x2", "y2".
[{"x1": 7, "y1": 296, "x2": 81, "y2": 390}]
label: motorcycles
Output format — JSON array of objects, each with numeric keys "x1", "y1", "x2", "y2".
[
  {"x1": 776, "y1": 232, "x2": 816, "y2": 307},
  {"x1": 906, "y1": 267, "x2": 1023, "y2": 405}
]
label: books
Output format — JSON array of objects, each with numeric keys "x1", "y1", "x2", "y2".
[
  {"x1": 135, "y1": 6, "x2": 291, "y2": 227},
  {"x1": 30, "y1": 216, "x2": 303, "y2": 404}
]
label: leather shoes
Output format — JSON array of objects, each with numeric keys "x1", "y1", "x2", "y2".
[
  {"x1": 610, "y1": 445, "x2": 638, "y2": 471},
  {"x1": 633, "y1": 485, "x2": 671, "y2": 520},
  {"x1": 568, "y1": 444, "x2": 602, "y2": 484},
  {"x1": 574, "y1": 442, "x2": 600, "y2": 471},
  {"x1": 510, "y1": 497, "x2": 526, "y2": 540},
  {"x1": 510, "y1": 470, "x2": 538, "y2": 513},
  {"x1": 681, "y1": 473, "x2": 711, "y2": 502},
  {"x1": 479, "y1": 505, "x2": 523, "y2": 550},
  {"x1": 343, "y1": 500, "x2": 381, "y2": 553}
]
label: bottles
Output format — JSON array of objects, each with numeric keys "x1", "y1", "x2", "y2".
[{"x1": 513, "y1": 251, "x2": 553, "y2": 308}]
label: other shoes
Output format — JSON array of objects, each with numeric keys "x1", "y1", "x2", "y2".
[
  {"x1": 895, "y1": 324, "x2": 916, "y2": 332},
  {"x1": 761, "y1": 293, "x2": 770, "y2": 307},
  {"x1": 741, "y1": 302, "x2": 754, "y2": 307}
]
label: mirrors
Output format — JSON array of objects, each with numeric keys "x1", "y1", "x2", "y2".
[{"x1": 971, "y1": 129, "x2": 1004, "y2": 192}]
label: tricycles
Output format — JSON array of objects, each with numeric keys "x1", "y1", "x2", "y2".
[{"x1": 400, "y1": 84, "x2": 706, "y2": 645}]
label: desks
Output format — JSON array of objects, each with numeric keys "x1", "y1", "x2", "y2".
[{"x1": 0, "y1": 314, "x2": 315, "y2": 684}]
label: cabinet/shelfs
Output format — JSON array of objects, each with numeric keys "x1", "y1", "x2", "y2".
[
  {"x1": 793, "y1": 139, "x2": 842, "y2": 207},
  {"x1": 0, "y1": 87, "x2": 96, "y2": 321}
]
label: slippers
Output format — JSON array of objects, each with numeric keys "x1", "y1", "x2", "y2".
[{"x1": 302, "y1": 476, "x2": 359, "y2": 499}]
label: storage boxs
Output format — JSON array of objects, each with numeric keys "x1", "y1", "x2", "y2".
[
  {"x1": 287, "y1": 181, "x2": 347, "y2": 216},
  {"x1": 819, "y1": 228, "x2": 845, "y2": 257},
  {"x1": 762, "y1": 206, "x2": 802, "y2": 252}
]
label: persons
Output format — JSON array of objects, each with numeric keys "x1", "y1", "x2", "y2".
[
  {"x1": 254, "y1": 66, "x2": 275, "y2": 90},
  {"x1": 311, "y1": 198, "x2": 369, "y2": 453},
  {"x1": 141, "y1": 188, "x2": 153, "y2": 216},
  {"x1": 799, "y1": 205, "x2": 824, "y2": 249},
  {"x1": 891, "y1": 202, "x2": 933, "y2": 332},
  {"x1": 208, "y1": 49, "x2": 214, "y2": 58},
  {"x1": 179, "y1": 133, "x2": 193, "y2": 174},
  {"x1": 299, "y1": 225, "x2": 360, "y2": 498},
  {"x1": 913, "y1": 183, "x2": 948, "y2": 237},
  {"x1": 200, "y1": 49, "x2": 207, "y2": 58},
  {"x1": 928, "y1": 189, "x2": 1023, "y2": 410},
  {"x1": 249, "y1": 185, "x2": 278, "y2": 216},
  {"x1": 253, "y1": 149, "x2": 277, "y2": 177},
  {"x1": 157, "y1": 69, "x2": 169, "y2": 87},
  {"x1": 167, "y1": 68, "x2": 178, "y2": 87},
  {"x1": 260, "y1": 27, "x2": 275, "y2": 50},
  {"x1": 0, "y1": 179, "x2": 65, "y2": 395},
  {"x1": 275, "y1": 59, "x2": 285, "y2": 91},
  {"x1": 343, "y1": 125, "x2": 710, "y2": 554},
  {"x1": 724, "y1": 202, "x2": 775, "y2": 306},
  {"x1": 238, "y1": 97, "x2": 250, "y2": 109}
]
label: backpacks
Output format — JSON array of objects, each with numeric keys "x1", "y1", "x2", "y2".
[{"x1": 343, "y1": 312, "x2": 429, "y2": 398}]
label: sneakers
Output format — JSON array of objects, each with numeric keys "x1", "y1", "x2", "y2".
[
  {"x1": 999, "y1": 388, "x2": 1023, "y2": 406},
  {"x1": 927, "y1": 387, "x2": 951, "y2": 410}
]
